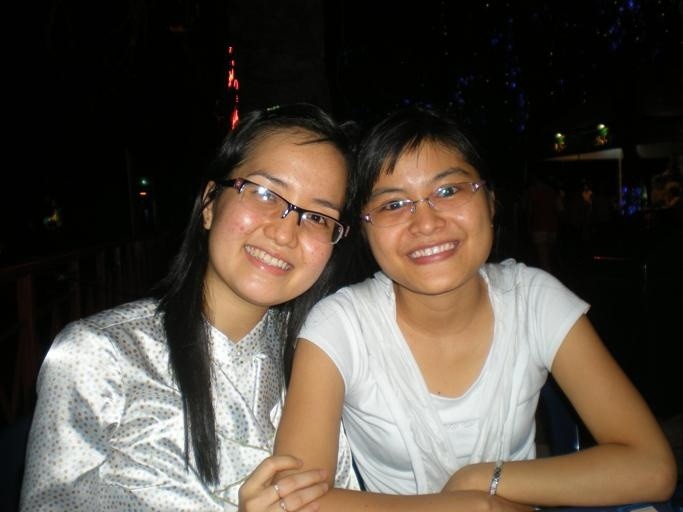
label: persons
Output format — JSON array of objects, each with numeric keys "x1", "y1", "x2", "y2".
[
  {"x1": 274, "y1": 108, "x2": 682, "y2": 512},
  {"x1": 17, "y1": 99, "x2": 360, "y2": 512}
]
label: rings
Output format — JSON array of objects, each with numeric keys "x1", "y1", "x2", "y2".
[
  {"x1": 271, "y1": 481, "x2": 281, "y2": 495},
  {"x1": 279, "y1": 500, "x2": 289, "y2": 510}
]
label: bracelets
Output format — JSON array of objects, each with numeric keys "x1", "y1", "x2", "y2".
[{"x1": 487, "y1": 459, "x2": 506, "y2": 497}]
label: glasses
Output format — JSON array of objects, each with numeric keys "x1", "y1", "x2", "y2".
[
  {"x1": 360, "y1": 180, "x2": 486, "y2": 229},
  {"x1": 221, "y1": 176, "x2": 350, "y2": 246}
]
